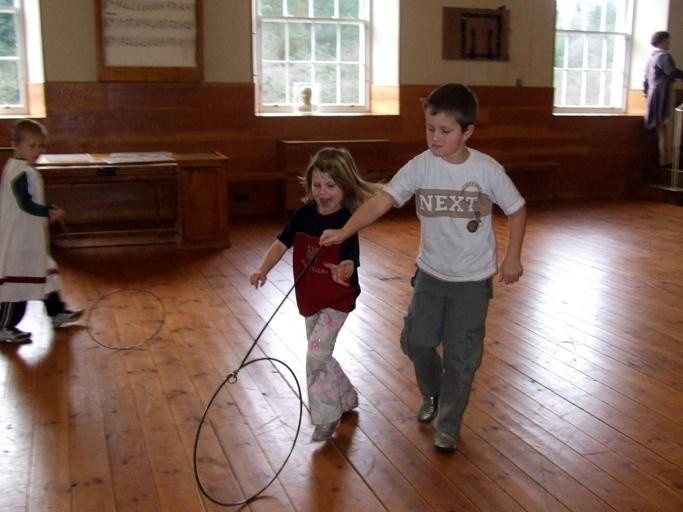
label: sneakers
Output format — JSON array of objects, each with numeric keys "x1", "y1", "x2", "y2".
[
  {"x1": 433, "y1": 412, "x2": 458, "y2": 451},
  {"x1": 0, "y1": 326, "x2": 32, "y2": 343},
  {"x1": 417, "y1": 368, "x2": 446, "y2": 423},
  {"x1": 312, "y1": 417, "x2": 341, "y2": 441},
  {"x1": 50, "y1": 309, "x2": 82, "y2": 328}
]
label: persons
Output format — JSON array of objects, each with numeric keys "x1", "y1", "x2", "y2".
[
  {"x1": 297, "y1": 87, "x2": 317, "y2": 110},
  {"x1": 249, "y1": 146, "x2": 384, "y2": 441},
  {"x1": 318, "y1": 82, "x2": 529, "y2": 451},
  {"x1": 641, "y1": 31, "x2": 683, "y2": 186},
  {"x1": 0, "y1": 119, "x2": 86, "y2": 345}
]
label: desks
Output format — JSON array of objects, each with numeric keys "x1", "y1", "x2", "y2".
[{"x1": 34, "y1": 148, "x2": 229, "y2": 251}]
